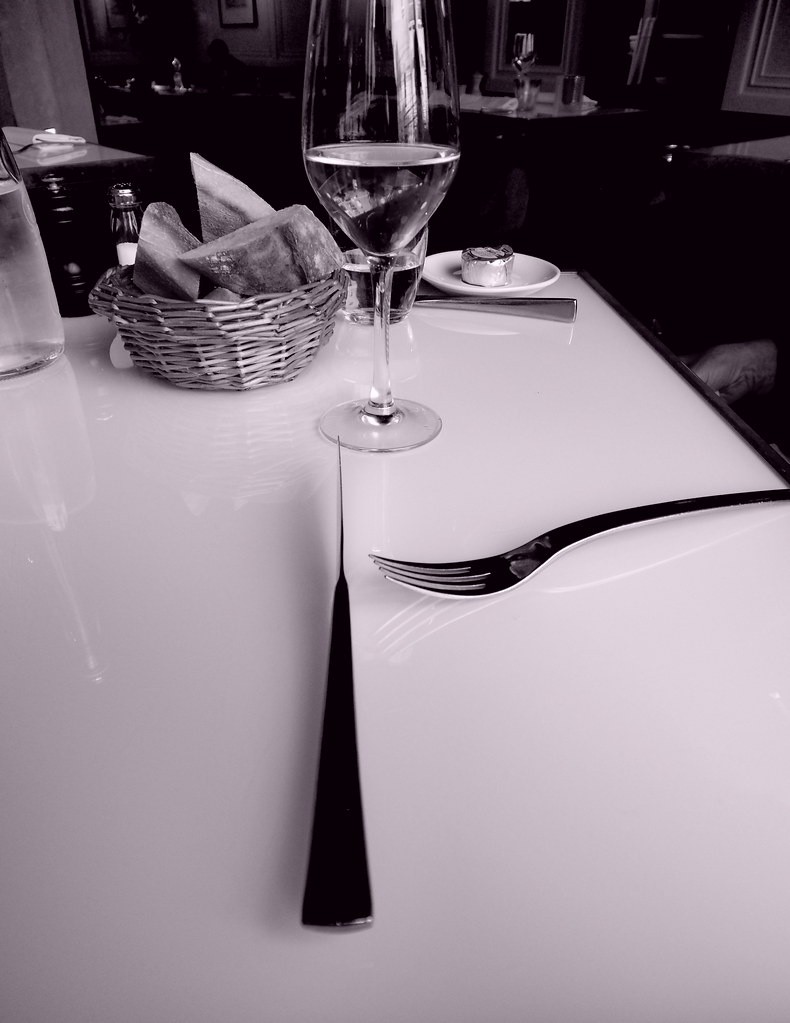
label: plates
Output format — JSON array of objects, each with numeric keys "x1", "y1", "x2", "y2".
[{"x1": 417, "y1": 250, "x2": 561, "y2": 297}]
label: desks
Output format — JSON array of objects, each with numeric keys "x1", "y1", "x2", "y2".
[
  {"x1": 1, "y1": 125, "x2": 158, "y2": 317},
  {"x1": 0, "y1": 263, "x2": 790, "y2": 1023},
  {"x1": 670, "y1": 125, "x2": 790, "y2": 189},
  {"x1": 376, "y1": 88, "x2": 650, "y2": 242}
]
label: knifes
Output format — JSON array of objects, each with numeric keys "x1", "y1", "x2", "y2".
[{"x1": 302, "y1": 436, "x2": 373, "y2": 928}]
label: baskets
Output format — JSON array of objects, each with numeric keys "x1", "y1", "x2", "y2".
[{"x1": 89, "y1": 263, "x2": 349, "y2": 392}]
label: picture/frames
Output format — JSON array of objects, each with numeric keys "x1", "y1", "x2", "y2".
[{"x1": 217, "y1": 0, "x2": 258, "y2": 28}]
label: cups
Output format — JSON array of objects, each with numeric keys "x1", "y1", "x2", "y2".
[
  {"x1": 555, "y1": 75, "x2": 585, "y2": 112},
  {"x1": 328, "y1": 214, "x2": 430, "y2": 326},
  {"x1": 513, "y1": 79, "x2": 542, "y2": 110}
]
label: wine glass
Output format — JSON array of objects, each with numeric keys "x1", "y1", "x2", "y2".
[
  {"x1": 513, "y1": 33, "x2": 538, "y2": 112},
  {"x1": 301, "y1": 0, "x2": 462, "y2": 455}
]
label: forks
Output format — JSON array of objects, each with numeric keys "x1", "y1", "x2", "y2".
[
  {"x1": 12, "y1": 141, "x2": 86, "y2": 154},
  {"x1": 368, "y1": 487, "x2": 790, "y2": 599}
]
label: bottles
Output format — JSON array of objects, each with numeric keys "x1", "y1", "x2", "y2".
[{"x1": 0, "y1": 125, "x2": 66, "y2": 381}]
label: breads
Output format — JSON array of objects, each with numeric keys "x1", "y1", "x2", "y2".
[{"x1": 132, "y1": 153, "x2": 344, "y2": 303}]
